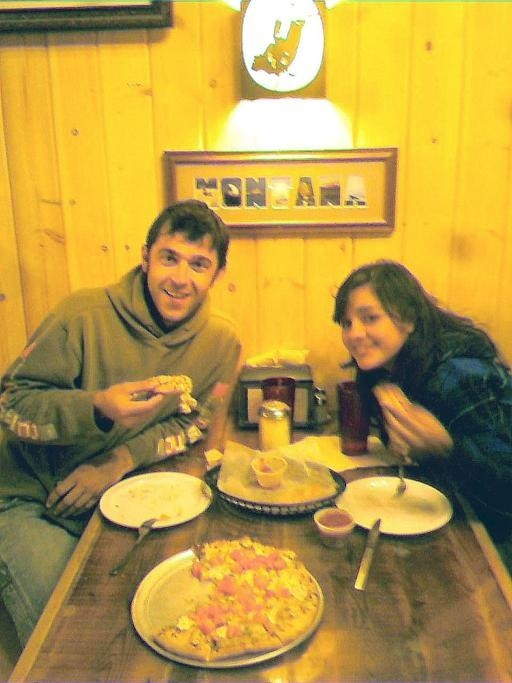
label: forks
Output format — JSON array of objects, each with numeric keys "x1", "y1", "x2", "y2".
[
  {"x1": 106, "y1": 516, "x2": 158, "y2": 575},
  {"x1": 390, "y1": 454, "x2": 409, "y2": 499}
]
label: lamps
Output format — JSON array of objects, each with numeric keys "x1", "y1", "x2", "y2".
[{"x1": 230, "y1": 0, "x2": 335, "y2": 98}]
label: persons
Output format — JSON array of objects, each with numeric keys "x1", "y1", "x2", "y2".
[
  {"x1": 332, "y1": 259, "x2": 512, "y2": 576},
  {"x1": 1, "y1": 200, "x2": 243, "y2": 652}
]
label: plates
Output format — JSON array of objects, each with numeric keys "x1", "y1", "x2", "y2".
[
  {"x1": 99, "y1": 472, "x2": 211, "y2": 530},
  {"x1": 205, "y1": 465, "x2": 348, "y2": 513},
  {"x1": 336, "y1": 476, "x2": 455, "y2": 537}
]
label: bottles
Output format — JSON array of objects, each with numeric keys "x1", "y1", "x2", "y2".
[
  {"x1": 256, "y1": 397, "x2": 291, "y2": 451},
  {"x1": 311, "y1": 383, "x2": 328, "y2": 425}
]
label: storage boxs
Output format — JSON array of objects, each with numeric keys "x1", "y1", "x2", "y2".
[{"x1": 229, "y1": 343, "x2": 328, "y2": 433}]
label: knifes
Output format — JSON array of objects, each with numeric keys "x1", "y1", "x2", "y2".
[{"x1": 353, "y1": 516, "x2": 382, "y2": 592}]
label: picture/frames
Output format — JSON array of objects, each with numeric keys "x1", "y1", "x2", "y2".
[
  {"x1": 160, "y1": 143, "x2": 404, "y2": 237},
  {"x1": 1, "y1": 1, "x2": 178, "y2": 37}
]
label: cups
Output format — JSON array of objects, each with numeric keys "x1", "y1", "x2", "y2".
[
  {"x1": 251, "y1": 456, "x2": 287, "y2": 488},
  {"x1": 261, "y1": 376, "x2": 297, "y2": 418},
  {"x1": 337, "y1": 380, "x2": 371, "y2": 455},
  {"x1": 313, "y1": 505, "x2": 356, "y2": 550}
]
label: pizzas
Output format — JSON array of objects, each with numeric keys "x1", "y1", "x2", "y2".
[
  {"x1": 146, "y1": 374, "x2": 198, "y2": 414},
  {"x1": 156, "y1": 536, "x2": 319, "y2": 661}
]
